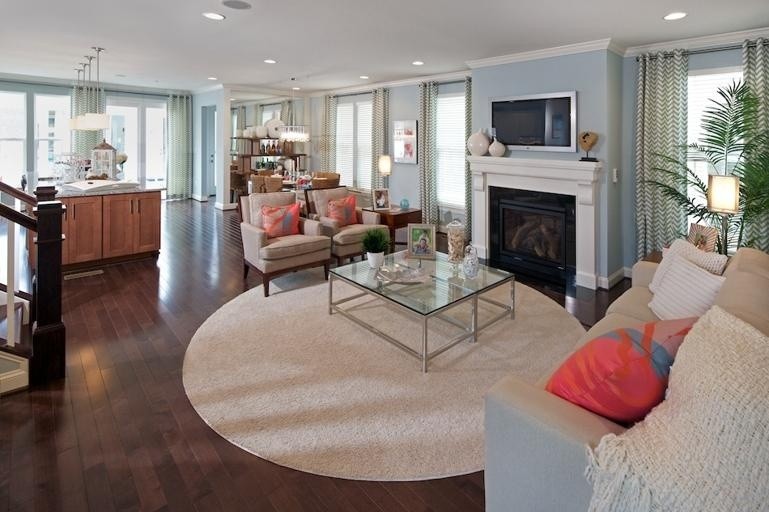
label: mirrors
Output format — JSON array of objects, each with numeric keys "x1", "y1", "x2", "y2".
[{"x1": 225, "y1": 83, "x2": 311, "y2": 202}]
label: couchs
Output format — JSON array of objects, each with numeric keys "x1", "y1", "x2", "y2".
[{"x1": 484, "y1": 247, "x2": 768, "y2": 511}]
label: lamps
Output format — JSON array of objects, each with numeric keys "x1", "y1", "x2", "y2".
[
  {"x1": 378, "y1": 155, "x2": 391, "y2": 189},
  {"x1": 708, "y1": 174, "x2": 739, "y2": 257},
  {"x1": 278, "y1": 77, "x2": 311, "y2": 143},
  {"x1": 68, "y1": 47, "x2": 109, "y2": 131}
]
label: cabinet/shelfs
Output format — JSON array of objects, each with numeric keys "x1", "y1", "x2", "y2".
[
  {"x1": 103, "y1": 191, "x2": 160, "y2": 268},
  {"x1": 24, "y1": 196, "x2": 104, "y2": 275},
  {"x1": 229, "y1": 137, "x2": 307, "y2": 203}
]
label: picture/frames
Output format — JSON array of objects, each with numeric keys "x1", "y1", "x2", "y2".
[
  {"x1": 687, "y1": 223, "x2": 718, "y2": 253},
  {"x1": 372, "y1": 189, "x2": 392, "y2": 211},
  {"x1": 408, "y1": 223, "x2": 436, "y2": 260},
  {"x1": 391, "y1": 120, "x2": 418, "y2": 165}
]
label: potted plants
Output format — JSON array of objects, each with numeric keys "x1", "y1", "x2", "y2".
[{"x1": 360, "y1": 226, "x2": 390, "y2": 268}]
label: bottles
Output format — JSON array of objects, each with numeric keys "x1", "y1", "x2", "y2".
[
  {"x1": 445, "y1": 219, "x2": 466, "y2": 264},
  {"x1": 257, "y1": 158, "x2": 268, "y2": 169},
  {"x1": 400, "y1": 199, "x2": 410, "y2": 210},
  {"x1": 466, "y1": 127, "x2": 506, "y2": 157},
  {"x1": 262, "y1": 140, "x2": 294, "y2": 155},
  {"x1": 464, "y1": 244, "x2": 480, "y2": 282}
]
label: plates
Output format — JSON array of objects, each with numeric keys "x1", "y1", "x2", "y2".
[
  {"x1": 372, "y1": 264, "x2": 435, "y2": 284},
  {"x1": 282, "y1": 181, "x2": 296, "y2": 185},
  {"x1": 271, "y1": 175, "x2": 282, "y2": 177}
]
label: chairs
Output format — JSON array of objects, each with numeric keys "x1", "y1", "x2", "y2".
[
  {"x1": 304, "y1": 186, "x2": 390, "y2": 267},
  {"x1": 240, "y1": 170, "x2": 341, "y2": 195},
  {"x1": 239, "y1": 190, "x2": 332, "y2": 298}
]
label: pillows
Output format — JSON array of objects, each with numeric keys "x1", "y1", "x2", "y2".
[
  {"x1": 312, "y1": 187, "x2": 358, "y2": 228},
  {"x1": 544, "y1": 239, "x2": 728, "y2": 424},
  {"x1": 247, "y1": 192, "x2": 302, "y2": 239}
]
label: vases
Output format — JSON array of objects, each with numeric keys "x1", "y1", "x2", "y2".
[{"x1": 466, "y1": 128, "x2": 505, "y2": 157}]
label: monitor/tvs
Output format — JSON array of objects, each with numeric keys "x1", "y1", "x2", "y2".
[{"x1": 489, "y1": 91, "x2": 576, "y2": 152}]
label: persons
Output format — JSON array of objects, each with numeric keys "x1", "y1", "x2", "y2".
[
  {"x1": 376, "y1": 191, "x2": 387, "y2": 208},
  {"x1": 417, "y1": 229, "x2": 430, "y2": 243},
  {"x1": 413, "y1": 239, "x2": 431, "y2": 255}
]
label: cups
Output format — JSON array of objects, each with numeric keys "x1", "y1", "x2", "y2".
[
  {"x1": 265, "y1": 162, "x2": 279, "y2": 168},
  {"x1": 274, "y1": 169, "x2": 279, "y2": 178},
  {"x1": 283, "y1": 170, "x2": 295, "y2": 180},
  {"x1": 242, "y1": 126, "x2": 268, "y2": 139},
  {"x1": 296, "y1": 170, "x2": 316, "y2": 186}
]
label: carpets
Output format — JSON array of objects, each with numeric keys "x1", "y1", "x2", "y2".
[{"x1": 181, "y1": 261, "x2": 587, "y2": 482}]
